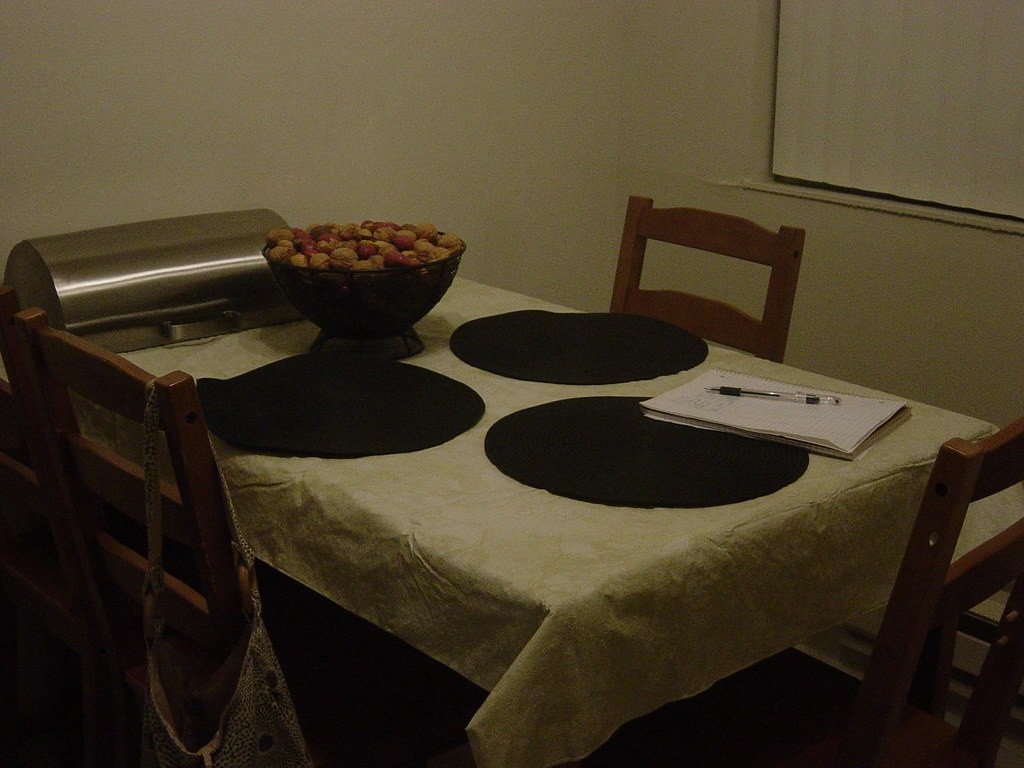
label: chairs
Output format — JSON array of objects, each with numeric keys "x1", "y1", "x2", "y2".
[
  {"x1": 0, "y1": 305, "x2": 244, "y2": 768},
  {"x1": 608, "y1": 194, "x2": 807, "y2": 364},
  {"x1": 777, "y1": 415, "x2": 1024, "y2": 768}
]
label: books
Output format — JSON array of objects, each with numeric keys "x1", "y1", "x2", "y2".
[{"x1": 640, "y1": 367, "x2": 912, "y2": 462}]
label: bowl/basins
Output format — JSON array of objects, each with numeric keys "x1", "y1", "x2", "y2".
[{"x1": 262, "y1": 242, "x2": 467, "y2": 358}]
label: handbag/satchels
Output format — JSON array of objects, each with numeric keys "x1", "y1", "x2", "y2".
[{"x1": 140, "y1": 377, "x2": 316, "y2": 768}]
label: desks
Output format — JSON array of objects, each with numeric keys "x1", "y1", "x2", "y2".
[{"x1": 118, "y1": 278, "x2": 1000, "y2": 768}]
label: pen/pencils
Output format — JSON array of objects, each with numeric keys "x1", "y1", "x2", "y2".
[{"x1": 705, "y1": 386, "x2": 842, "y2": 404}]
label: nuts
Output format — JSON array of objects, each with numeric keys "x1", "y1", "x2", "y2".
[{"x1": 264, "y1": 220, "x2": 464, "y2": 286}]
